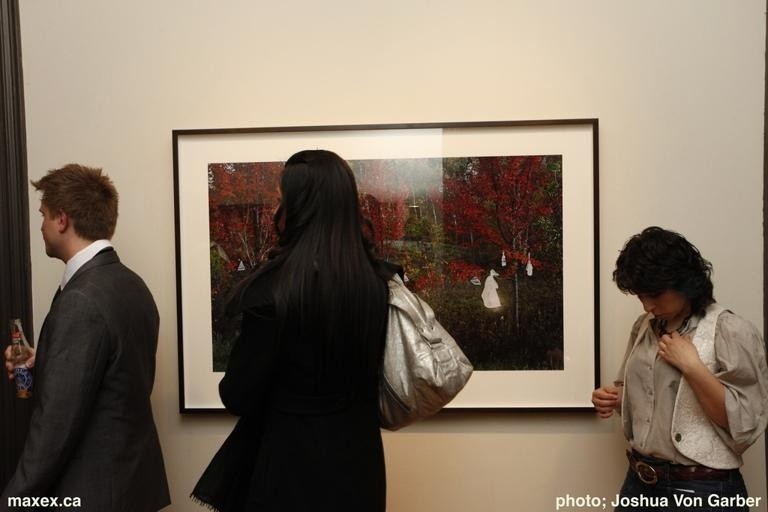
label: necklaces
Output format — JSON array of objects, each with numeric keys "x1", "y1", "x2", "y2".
[{"x1": 657, "y1": 317, "x2": 689, "y2": 337}]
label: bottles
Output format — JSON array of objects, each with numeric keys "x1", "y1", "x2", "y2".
[{"x1": 9, "y1": 318, "x2": 34, "y2": 400}]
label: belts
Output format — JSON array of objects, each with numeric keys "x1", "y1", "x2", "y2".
[{"x1": 624, "y1": 450, "x2": 712, "y2": 489}]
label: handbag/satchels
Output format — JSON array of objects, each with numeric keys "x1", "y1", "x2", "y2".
[{"x1": 373, "y1": 266, "x2": 475, "y2": 437}]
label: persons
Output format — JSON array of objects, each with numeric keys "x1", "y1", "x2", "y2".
[
  {"x1": 590, "y1": 224, "x2": 767, "y2": 512},
  {"x1": 189, "y1": 148, "x2": 390, "y2": 512},
  {"x1": 0, "y1": 163, "x2": 171, "y2": 512}
]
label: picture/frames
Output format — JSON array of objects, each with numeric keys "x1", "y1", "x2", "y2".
[{"x1": 172, "y1": 118, "x2": 602, "y2": 414}]
